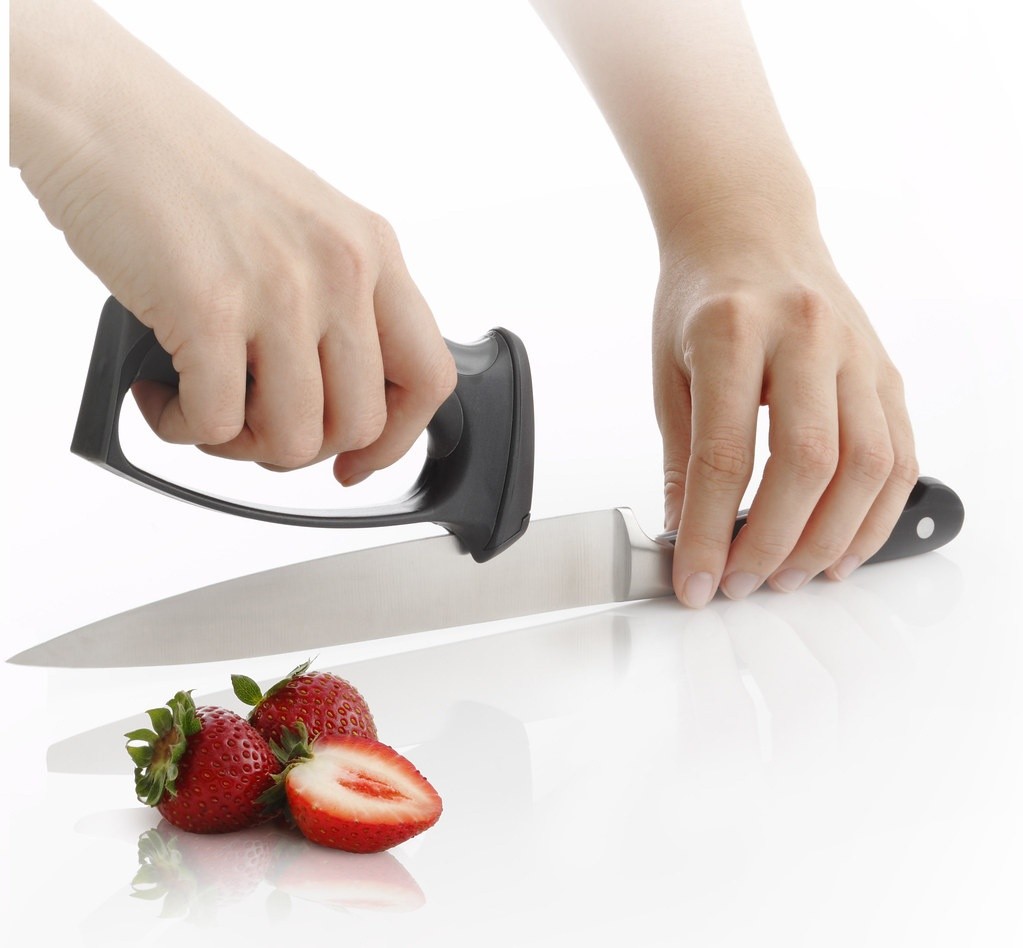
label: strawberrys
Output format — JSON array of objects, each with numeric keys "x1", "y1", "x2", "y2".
[{"x1": 124, "y1": 652, "x2": 443, "y2": 854}]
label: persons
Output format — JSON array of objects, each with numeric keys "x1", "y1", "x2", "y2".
[{"x1": 9, "y1": 0, "x2": 919, "y2": 612}]
